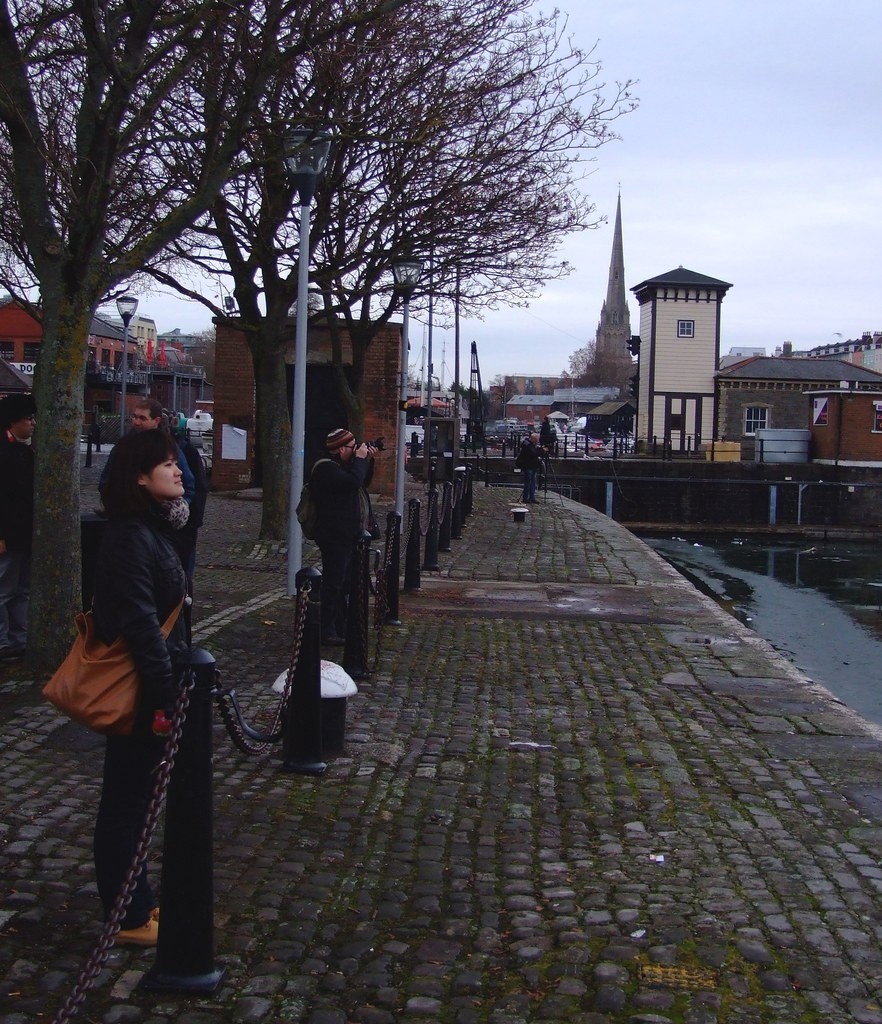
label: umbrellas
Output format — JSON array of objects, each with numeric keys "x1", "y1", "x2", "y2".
[
  {"x1": 547, "y1": 411, "x2": 570, "y2": 419},
  {"x1": 147, "y1": 338, "x2": 167, "y2": 370}
]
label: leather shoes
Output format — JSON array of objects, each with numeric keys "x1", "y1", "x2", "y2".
[
  {"x1": 111, "y1": 921, "x2": 158, "y2": 946},
  {"x1": 149, "y1": 908, "x2": 160, "y2": 923}
]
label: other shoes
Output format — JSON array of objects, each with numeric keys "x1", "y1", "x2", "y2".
[
  {"x1": 529, "y1": 499, "x2": 539, "y2": 504},
  {"x1": 0, "y1": 646, "x2": 26, "y2": 661},
  {"x1": 523, "y1": 500, "x2": 530, "y2": 504},
  {"x1": 320, "y1": 633, "x2": 345, "y2": 645}
]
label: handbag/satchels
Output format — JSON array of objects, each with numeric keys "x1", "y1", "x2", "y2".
[{"x1": 43, "y1": 611, "x2": 143, "y2": 736}]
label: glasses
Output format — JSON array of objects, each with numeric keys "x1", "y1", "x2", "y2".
[{"x1": 344, "y1": 444, "x2": 356, "y2": 452}]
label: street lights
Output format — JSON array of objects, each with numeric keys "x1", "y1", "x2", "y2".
[
  {"x1": 280, "y1": 128, "x2": 333, "y2": 598},
  {"x1": 117, "y1": 295, "x2": 139, "y2": 439},
  {"x1": 390, "y1": 257, "x2": 424, "y2": 534}
]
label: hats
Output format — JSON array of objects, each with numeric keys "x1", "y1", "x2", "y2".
[
  {"x1": 326, "y1": 429, "x2": 354, "y2": 449},
  {"x1": 0, "y1": 394, "x2": 37, "y2": 432}
]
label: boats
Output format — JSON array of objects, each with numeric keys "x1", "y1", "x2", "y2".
[{"x1": 486, "y1": 417, "x2": 635, "y2": 452}]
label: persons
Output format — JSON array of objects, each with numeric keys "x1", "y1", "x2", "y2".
[
  {"x1": 515, "y1": 433, "x2": 549, "y2": 503},
  {"x1": 539, "y1": 417, "x2": 555, "y2": 456},
  {"x1": 80, "y1": 425, "x2": 204, "y2": 944},
  {"x1": 0, "y1": 393, "x2": 38, "y2": 658},
  {"x1": 312, "y1": 429, "x2": 377, "y2": 646},
  {"x1": 98, "y1": 399, "x2": 195, "y2": 697},
  {"x1": 176, "y1": 439, "x2": 209, "y2": 647}
]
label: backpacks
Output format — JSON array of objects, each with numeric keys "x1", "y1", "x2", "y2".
[
  {"x1": 515, "y1": 445, "x2": 534, "y2": 468},
  {"x1": 296, "y1": 458, "x2": 342, "y2": 540}
]
